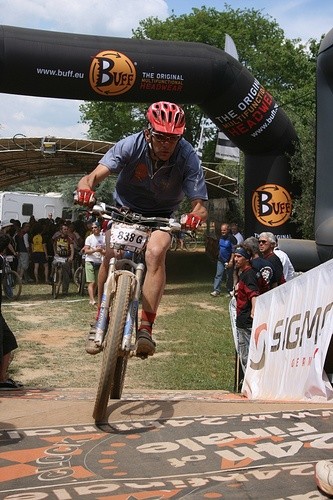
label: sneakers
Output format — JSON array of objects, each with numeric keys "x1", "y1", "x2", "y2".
[
  {"x1": 211, "y1": 291, "x2": 220, "y2": 296},
  {"x1": 7, "y1": 379, "x2": 25, "y2": 389},
  {"x1": 226, "y1": 294, "x2": 230, "y2": 298},
  {"x1": 86, "y1": 320, "x2": 105, "y2": 354},
  {"x1": 136, "y1": 332, "x2": 156, "y2": 355},
  {"x1": 314, "y1": 461, "x2": 333, "y2": 496},
  {"x1": 0, "y1": 381, "x2": 21, "y2": 390}
]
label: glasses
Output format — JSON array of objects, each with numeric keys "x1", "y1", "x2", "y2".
[
  {"x1": 258, "y1": 240, "x2": 267, "y2": 244},
  {"x1": 150, "y1": 130, "x2": 179, "y2": 143},
  {"x1": 64, "y1": 228, "x2": 69, "y2": 230},
  {"x1": 92, "y1": 227, "x2": 97, "y2": 229}
]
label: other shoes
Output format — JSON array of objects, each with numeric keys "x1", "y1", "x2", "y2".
[{"x1": 92, "y1": 302, "x2": 99, "y2": 310}]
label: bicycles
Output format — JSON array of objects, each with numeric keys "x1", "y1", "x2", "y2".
[
  {"x1": 170, "y1": 236, "x2": 198, "y2": 251},
  {"x1": 0, "y1": 250, "x2": 90, "y2": 301},
  {"x1": 70, "y1": 191, "x2": 201, "y2": 424}
]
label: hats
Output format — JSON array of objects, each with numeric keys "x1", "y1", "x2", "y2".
[{"x1": 92, "y1": 222, "x2": 99, "y2": 227}]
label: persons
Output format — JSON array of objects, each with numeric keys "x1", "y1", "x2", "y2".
[
  {"x1": 230, "y1": 223, "x2": 244, "y2": 244},
  {"x1": 230, "y1": 232, "x2": 294, "y2": 378},
  {"x1": 0, "y1": 216, "x2": 196, "y2": 300},
  {"x1": 210, "y1": 223, "x2": 237, "y2": 297},
  {"x1": 73, "y1": 102, "x2": 208, "y2": 357},
  {"x1": 315, "y1": 459, "x2": 333, "y2": 495},
  {"x1": 85, "y1": 222, "x2": 106, "y2": 305},
  {"x1": 0, "y1": 312, "x2": 23, "y2": 390}
]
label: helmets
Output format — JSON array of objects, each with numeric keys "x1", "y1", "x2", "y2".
[{"x1": 145, "y1": 101, "x2": 185, "y2": 134}]
